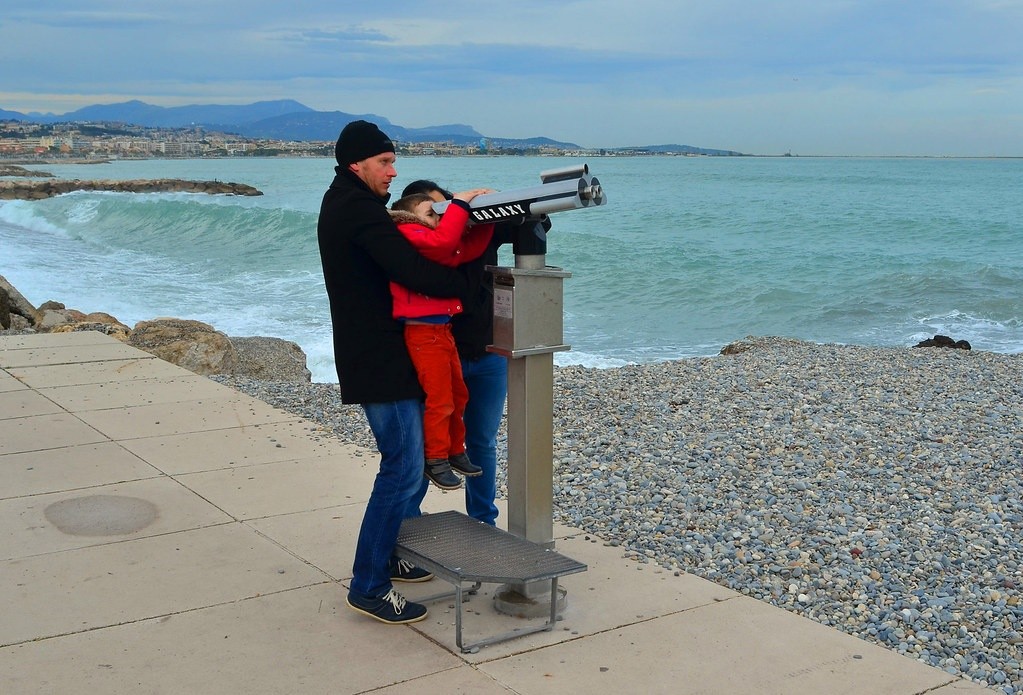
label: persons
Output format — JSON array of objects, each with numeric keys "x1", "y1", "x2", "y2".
[
  {"x1": 400, "y1": 179, "x2": 552, "y2": 528},
  {"x1": 315, "y1": 121, "x2": 484, "y2": 624},
  {"x1": 386, "y1": 189, "x2": 498, "y2": 491}
]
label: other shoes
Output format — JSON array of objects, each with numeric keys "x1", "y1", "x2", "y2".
[
  {"x1": 448, "y1": 453, "x2": 482, "y2": 477},
  {"x1": 424, "y1": 461, "x2": 462, "y2": 490}
]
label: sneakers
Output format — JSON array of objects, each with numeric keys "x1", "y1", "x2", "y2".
[
  {"x1": 346, "y1": 588, "x2": 428, "y2": 625},
  {"x1": 390, "y1": 558, "x2": 435, "y2": 583}
]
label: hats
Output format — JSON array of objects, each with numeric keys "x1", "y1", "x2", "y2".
[{"x1": 335, "y1": 120, "x2": 395, "y2": 165}]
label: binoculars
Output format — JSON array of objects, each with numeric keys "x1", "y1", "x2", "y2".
[{"x1": 431, "y1": 163, "x2": 609, "y2": 229}]
